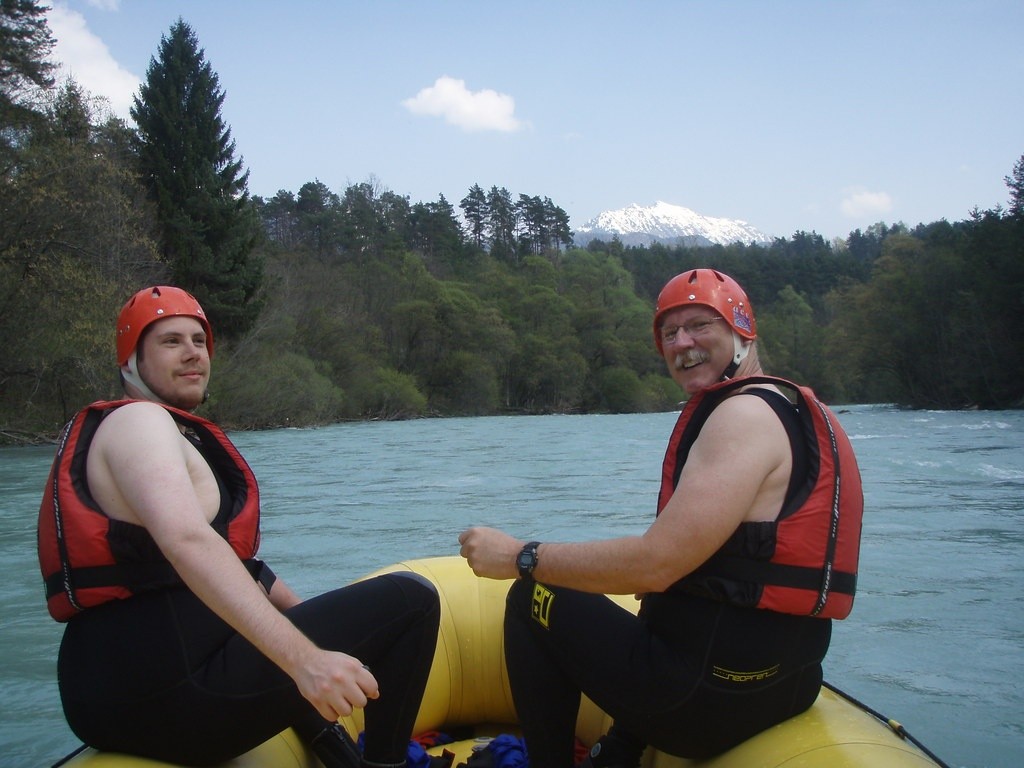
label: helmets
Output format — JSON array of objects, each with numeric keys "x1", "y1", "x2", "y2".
[
  {"x1": 115, "y1": 286, "x2": 214, "y2": 367},
  {"x1": 651, "y1": 268, "x2": 757, "y2": 348}
]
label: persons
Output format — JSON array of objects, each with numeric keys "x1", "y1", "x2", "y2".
[
  {"x1": 37, "y1": 286, "x2": 441, "y2": 768},
  {"x1": 458, "y1": 269, "x2": 864, "y2": 768}
]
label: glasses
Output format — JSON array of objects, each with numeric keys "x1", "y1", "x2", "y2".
[{"x1": 660, "y1": 316, "x2": 724, "y2": 344}]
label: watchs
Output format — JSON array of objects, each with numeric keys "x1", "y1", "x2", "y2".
[{"x1": 516, "y1": 542, "x2": 543, "y2": 580}]
